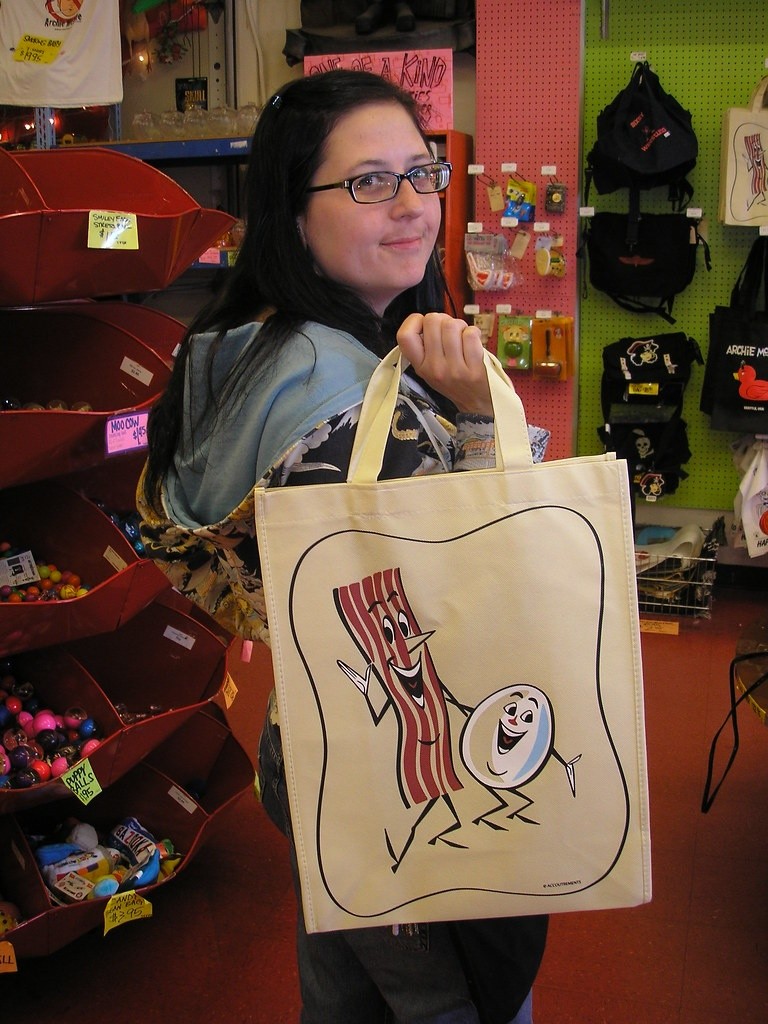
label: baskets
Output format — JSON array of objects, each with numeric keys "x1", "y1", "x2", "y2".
[{"x1": 634, "y1": 554, "x2": 715, "y2": 620}]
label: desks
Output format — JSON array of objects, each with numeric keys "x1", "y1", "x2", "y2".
[{"x1": 729, "y1": 612, "x2": 768, "y2": 726}]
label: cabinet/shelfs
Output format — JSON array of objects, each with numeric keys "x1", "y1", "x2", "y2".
[
  {"x1": 423, "y1": 129, "x2": 474, "y2": 321},
  {"x1": 33, "y1": 106, "x2": 251, "y2": 268}
]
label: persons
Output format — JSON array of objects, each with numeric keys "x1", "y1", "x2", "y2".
[{"x1": 135, "y1": 70, "x2": 551, "y2": 1024}]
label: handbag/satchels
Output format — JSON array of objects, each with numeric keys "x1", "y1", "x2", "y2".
[
  {"x1": 253, "y1": 329, "x2": 651, "y2": 934},
  {"x1": 572, "y1": 65, "x2": 768, "y2": 559}
]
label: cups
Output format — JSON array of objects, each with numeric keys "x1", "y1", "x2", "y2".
[{"x1": 131, "y1": 103, "x2": 260, "y2": 139}]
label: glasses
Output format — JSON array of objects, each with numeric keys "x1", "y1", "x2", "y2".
[{"x1": 305, "y1": 161, "x2": 453, "y2": 204}]
